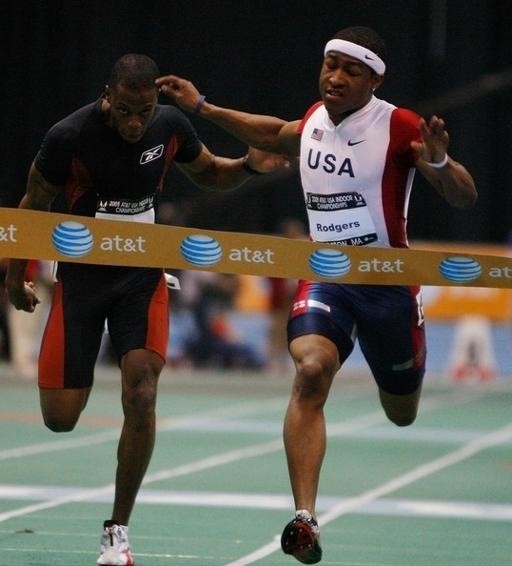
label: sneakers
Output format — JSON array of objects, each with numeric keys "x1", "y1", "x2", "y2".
[
  {"x1": 278, "y1": 513, "x2": 326, "y2": 562},
  {"x1": 92, "y1": 521, "x2": 135, "y2": 561}
]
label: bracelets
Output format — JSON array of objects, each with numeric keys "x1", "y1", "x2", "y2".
[{"x1": 194, "y1": 94, "x2": 209, "y2": 115}]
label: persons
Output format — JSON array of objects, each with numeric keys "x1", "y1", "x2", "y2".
[
  {"x1": 4, "y1": 52, "x2": 294, "y2": 565},
  {"x1": 147, "y1": 22, "x2": 484, "y2": 566},
  {"x1": 0, "y1": 151, "x2": 511, "y2": 384}
]
